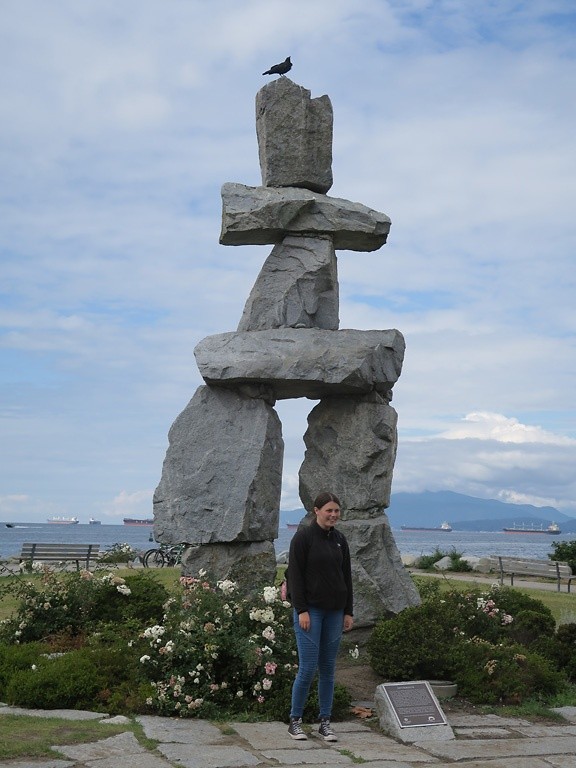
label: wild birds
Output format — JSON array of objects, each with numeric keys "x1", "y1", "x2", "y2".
[{"x1": 262, "y1": 57, "x2": 294, "y2": 78}]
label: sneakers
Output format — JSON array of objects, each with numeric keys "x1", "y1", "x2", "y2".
[
  {"x1": 287, "y1": 721, "x2": 308, "y2": 740},
  {"x1": 318, "y1": 717, "x2": 337, "y2": 741}
]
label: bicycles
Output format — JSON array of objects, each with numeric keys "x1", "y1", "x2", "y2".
[
  {"x1": 142, "y1": 543, "x2": 185, "y2": 569},
  {"x1": 146, "y1": 543, "x2": 185, "y2": 570}
]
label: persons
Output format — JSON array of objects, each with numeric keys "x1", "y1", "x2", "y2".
[{"x1": 287, "y1": 492, "x2": 353, "y2": 741}]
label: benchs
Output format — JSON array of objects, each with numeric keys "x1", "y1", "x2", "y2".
[
  {"x1": 489, "y1": 556, "x2": 576, "y2": 593},
  {"x1": 10, "y1": 542, "x2": 100, "y2": 575}
]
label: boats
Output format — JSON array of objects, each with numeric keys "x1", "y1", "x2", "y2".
[
  {"x1": 399, "y1": 520, "x2": 451, "y2": 532},
  {"x1": 88, "y1": 517, "x2": 101, "y2": 526},
  {"x1": 286, "y1": 523, "x2": 299, "y2": 529},
  {"x1": 503, "y1": 521, "x2": 561, "y2": 535},
  {"x1": 47, "y1": 515, "x2": 79, "y2": 525},
  {"x1": 123, "y1": 517, "x2": 155, "y2": 526},
  {"x1": 5, "y1": 523, "x2": 15, "y2": 528}
]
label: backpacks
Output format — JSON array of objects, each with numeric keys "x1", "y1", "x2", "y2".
[{"x1": 279, "y1": 527, "x2": 344, "y2": 609}]
